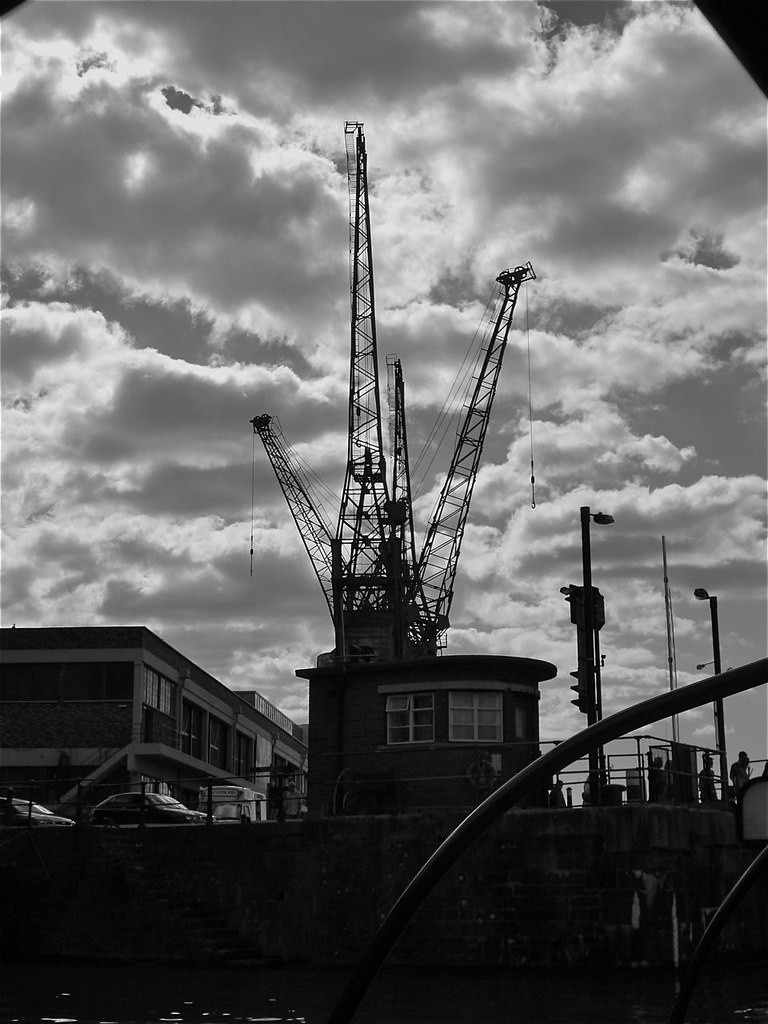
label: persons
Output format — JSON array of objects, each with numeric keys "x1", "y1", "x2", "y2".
[
  {"x1": 230, "y1": 791, "x2": 254, "y2": 824},
  {"x1": 653, "y1": 757, "x2": 673, "y2": 788},
  {"x1": 698, "y1": 757, "x2": 717, "y2": 803},
  {"x1": 549, "y1": 780, "x2": 566, "y2": 808},
  {"x1": 729, "y1": 752, "x2": 750, "y2": 799},
  {"x1": 582, "y1": 775, "x2": 592, "y2": 807},
  {"x1": 266, "y1": 782, "x2": 302, "y2": 821}
]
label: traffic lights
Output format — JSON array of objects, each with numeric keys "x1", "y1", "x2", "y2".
[{"x1": 569, "y1": 667, "x2": 588, "y2": 715}]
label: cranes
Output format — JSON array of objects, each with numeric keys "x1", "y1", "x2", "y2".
[{"x1": 250, "y1": 120, "x2": 539, "y2": 662}]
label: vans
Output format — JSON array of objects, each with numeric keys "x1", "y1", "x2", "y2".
[{"x1": 195, "y1": 785, "x2": 267, "y2": 823}]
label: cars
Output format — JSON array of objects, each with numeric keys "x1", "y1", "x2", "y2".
[
  {"x1": 0, "y1": 797, "x2": 76, "y2": 828},
  {"x1": 88, "y1": 790, "x2": 218, "y2": 824}
]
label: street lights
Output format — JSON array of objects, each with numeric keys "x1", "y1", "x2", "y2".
[
  {"x1": 577, "y1": 504, "x2": 617, "y2": 810},
  {"x1": 692, "y1": 587, "x2": 730, "y2": 801}
]
